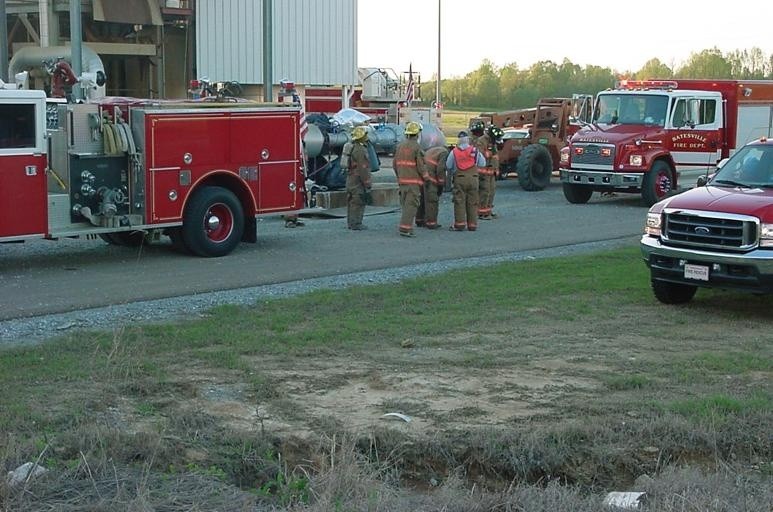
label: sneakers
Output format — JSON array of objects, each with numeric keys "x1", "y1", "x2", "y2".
[
  {"x1": 399, "y1": 231, "x2": 417, "y2": 238},
  {"x1": 416, "y1": 222, "x2": 478, "y2": 231},
  {"x1": 348, "y1": 224, "x2": 367, "y2": 231},
  {"x1": 478, "y1": 214, "x2": 499, "y2": 220}
]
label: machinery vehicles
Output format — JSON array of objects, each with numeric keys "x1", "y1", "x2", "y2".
[{"x1": 466, "y1": 92, "x2": 600, "y2": 194}]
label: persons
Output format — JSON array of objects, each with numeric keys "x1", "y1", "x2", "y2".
[
  {"x1": 346, "y1": 129, "x2": 372, "y2": 230},
  {"x1": 285, "y1": 213, "x2": 305, "y2": 228},
  {"x1": 393, "y1": 121, "x2": 429, "y2": 237},
  {"x1": 414, "y1": 121, "x2": 504, "y2": 232}
]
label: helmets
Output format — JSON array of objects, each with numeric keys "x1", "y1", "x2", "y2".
[
  {"x1": 350, "y1": 127, "x2": 367, "y2": 142},
  {"x1": 469, "y1": 118, "x2": 484, "y2": 131},
  {"x1": 488, "y1": 124, "x2": 504, "y2": 138},
  {"x1": 403, "y1": 121, "x2": 422, "y2": 135},
  {"x1": 457, "y1": 131, "x2": 468, "y2": 138}
]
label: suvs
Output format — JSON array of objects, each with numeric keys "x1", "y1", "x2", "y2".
[{"x1": 638, "y1": 136, "x2": 773, "y2": 306}]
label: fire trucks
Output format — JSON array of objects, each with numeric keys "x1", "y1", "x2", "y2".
[
  {"x1": 0, "y1": 59, "x2": 304, "y2": 256},
  {"x1": 555, "y1": 78, "x2": 772, "y2": 206},
  {"x1": 305, "y1": 69, "x2": 443, "y2": 155}
]
